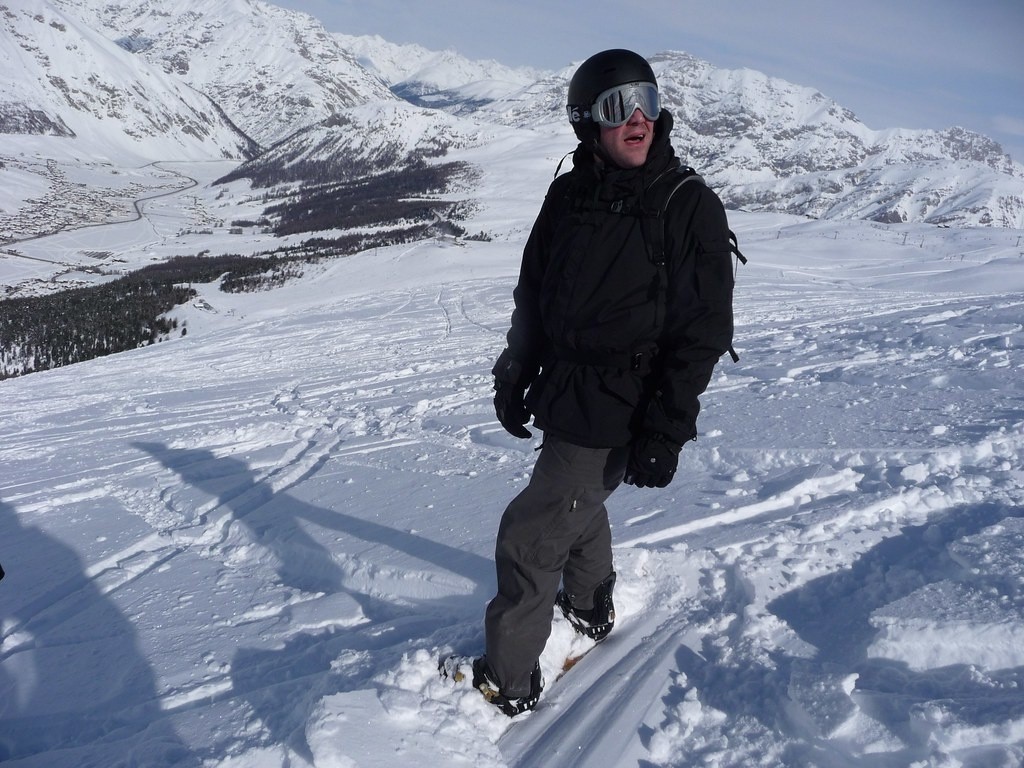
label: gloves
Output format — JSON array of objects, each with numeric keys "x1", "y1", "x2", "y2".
[
  {"x1": 493, "y1": 378, "x2": 533, "y2": 439},
  {"x1": 623, "y1": 428, "x2": 682, "y2": 488}
]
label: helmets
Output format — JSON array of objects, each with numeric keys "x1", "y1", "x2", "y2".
[{"x1": 567, "y1": 49, "x2": 657, "y2": 142}]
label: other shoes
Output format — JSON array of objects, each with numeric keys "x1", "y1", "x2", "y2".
[
  {"x1": 554, "y1": 572, "x2": 616, "y2": 642},
  {"x1": 440, "y1": 652, "x2": 544, "y2": 717}
]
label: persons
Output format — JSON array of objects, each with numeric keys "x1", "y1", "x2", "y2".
[{"x1": 435, "y1": 48, "x2": 736, "y2": 718}]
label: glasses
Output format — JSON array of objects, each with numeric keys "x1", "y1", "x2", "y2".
[{"x1": 565, "y1": 83, "x2": 663, "y2": 126}]
